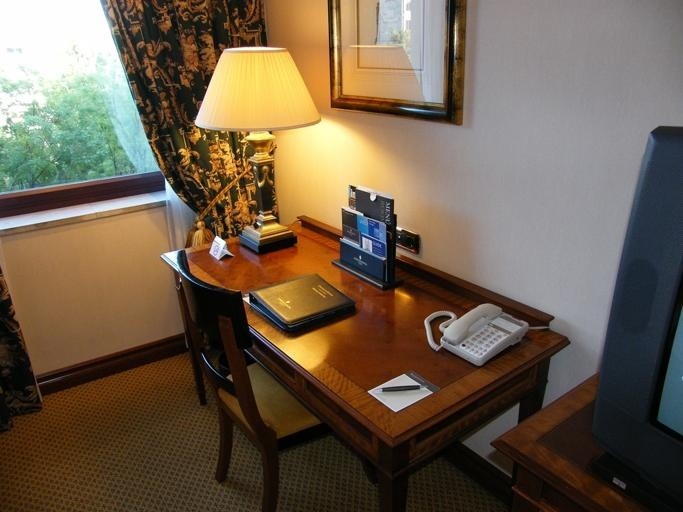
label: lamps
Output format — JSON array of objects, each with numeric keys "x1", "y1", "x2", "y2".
[
  {"x1": 193, "y1": 45, "x2": 322, "y2": 254},
  {"x1": 340, "y1": 43, "x2": 427, "y2": 103}
]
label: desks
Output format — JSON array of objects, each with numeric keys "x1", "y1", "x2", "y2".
[
  {"x1": 490, "y1": 371, "x2": 656, "y2": 511},
  {"x1": 159, "y1": 215, "x2": 572, "y2": 511}
]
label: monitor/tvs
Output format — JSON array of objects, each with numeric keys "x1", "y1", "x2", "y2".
[{"x1": 590, "y1": 125, "x2": 683, "y2": 512}]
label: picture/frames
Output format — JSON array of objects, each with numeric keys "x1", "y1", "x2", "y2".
[{"x1": 328, "y1": 2, "x2": 466, "y2": 126}]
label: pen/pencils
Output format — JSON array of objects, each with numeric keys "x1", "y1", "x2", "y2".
[{"x1": 380, "y1": 385, "x2": 428, "y2": 392}]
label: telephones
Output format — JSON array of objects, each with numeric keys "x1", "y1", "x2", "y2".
[{"x1": 440, "y1": 304, "x2": 529, "y2": 367}]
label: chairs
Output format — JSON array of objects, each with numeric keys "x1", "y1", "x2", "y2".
[{"x1": 175, "y1": 245, "x2": 332, "y2": 511}]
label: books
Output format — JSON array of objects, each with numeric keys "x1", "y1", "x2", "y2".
[{"x1": 338, "y1": 183, "x2": 399, "y2": 282}]
